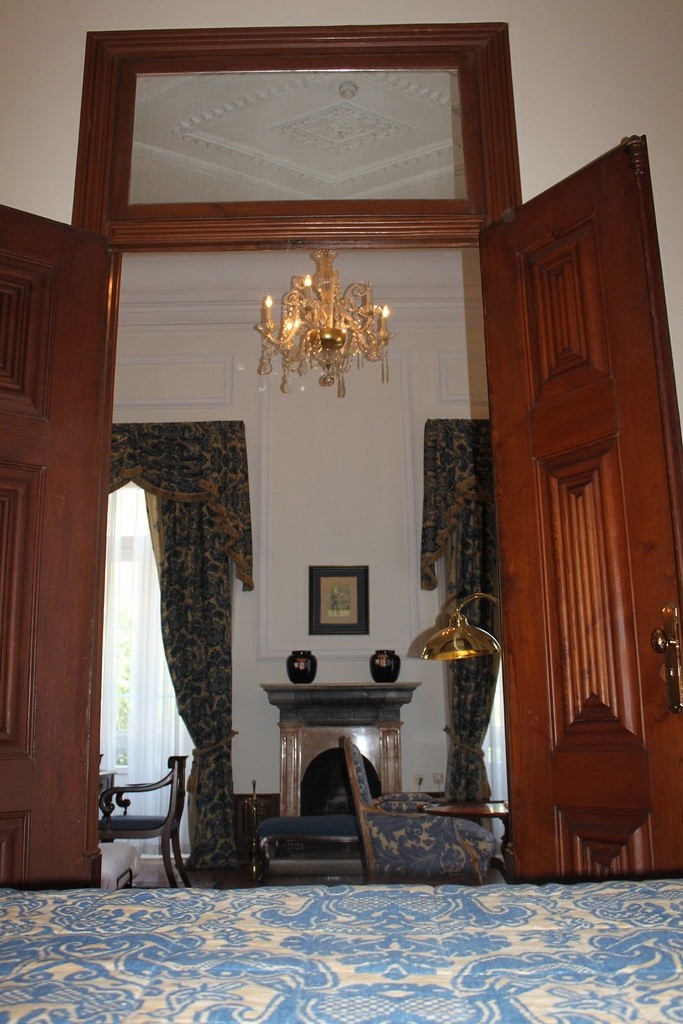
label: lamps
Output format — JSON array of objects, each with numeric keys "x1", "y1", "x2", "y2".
[
  {"x1": 254, "y1": 245, "x2": 393, "y2": 398},
  {"x1": 421, "y1": 593, "x2": 501, "y2": 661}
]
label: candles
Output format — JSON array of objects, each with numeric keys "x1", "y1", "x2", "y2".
[{"x1": 251, "y1": 778, "x2": 257, "y2": 800}]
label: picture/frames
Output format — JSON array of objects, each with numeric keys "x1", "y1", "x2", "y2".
[{"x1": 307, "y1": 565, "x2": 370, "y2": 636}]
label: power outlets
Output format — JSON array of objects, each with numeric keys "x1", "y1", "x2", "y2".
[
  {"x1": 433, "y1": 772, "x2": 443, "y2": 784},
  {"x1": 414, "y1": 773, "x2": 426, "y2": 785}
]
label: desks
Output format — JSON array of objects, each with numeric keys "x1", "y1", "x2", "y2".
[
  {"x1": 98, "y1": 768, "x2": 117, "y2": 796},
  {"x1": 424, "y1": 801, "x2": 510, "y2": 862}
]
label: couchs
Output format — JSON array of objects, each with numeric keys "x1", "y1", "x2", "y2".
[
  {"x1": 339, "y1": 735, "x2": 499, "y2": 884},
  {"x1": 252, "y1": 814, "x2": 367, "y2": 886}
]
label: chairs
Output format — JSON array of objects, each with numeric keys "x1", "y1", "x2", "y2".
[{"x1": 99, "y1": 756, "x2": 190, "y2": 888}]
